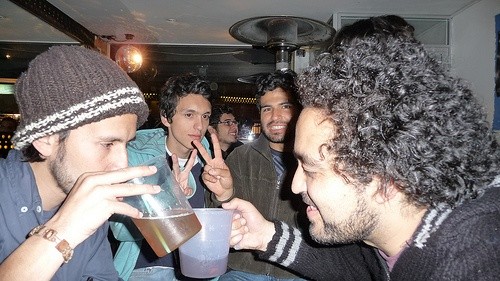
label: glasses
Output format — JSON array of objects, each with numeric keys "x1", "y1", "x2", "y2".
[{"x1": 211, "y1": 120, "x2": 239, "y2": 126}]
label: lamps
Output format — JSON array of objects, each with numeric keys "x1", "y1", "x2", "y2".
[{"x1": 115, "y1": 34, "x2": 142, "y2": 73}]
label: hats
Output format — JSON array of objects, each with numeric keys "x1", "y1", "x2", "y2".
[{"x1": 11, "y1": 44, "x2": 150, "y2": 151}]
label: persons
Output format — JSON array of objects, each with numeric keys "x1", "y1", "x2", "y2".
[
  {"x1": 0, "y1": 45, "x2": 161, "y2": 281},
  {"x1": 109, "y1": 71, "x2": 213, "y2": 281},
  {"x1": 0, "y1": 117, "x2": 18, "y2": 159},
  {"x1": 193, "y1": 68, "x2": 305, "y2": 281},
  {"x1": 136, "y1": 99, "x2": 162, "y2": 130},
  {"x1": 333, "y1": 14, "x2": 415, "y2": 39},
  {"x1": 204, "y1": 105, "x2": 256, "y2": 161},
  {"x1": 222, "y1": 34, "x2": 500, "y2": 281}
]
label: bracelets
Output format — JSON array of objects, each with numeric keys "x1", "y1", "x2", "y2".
[{"x1": 27, "y1": 224, "x2": 74, "y2": 263}]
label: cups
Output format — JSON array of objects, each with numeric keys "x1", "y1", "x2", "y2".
[
  {"x1": 178, "y1": 207, "x2": 233, "y2": 278},
  {"x1": 114, "y1": 154, "x2": 202, "y2": 257}
]
